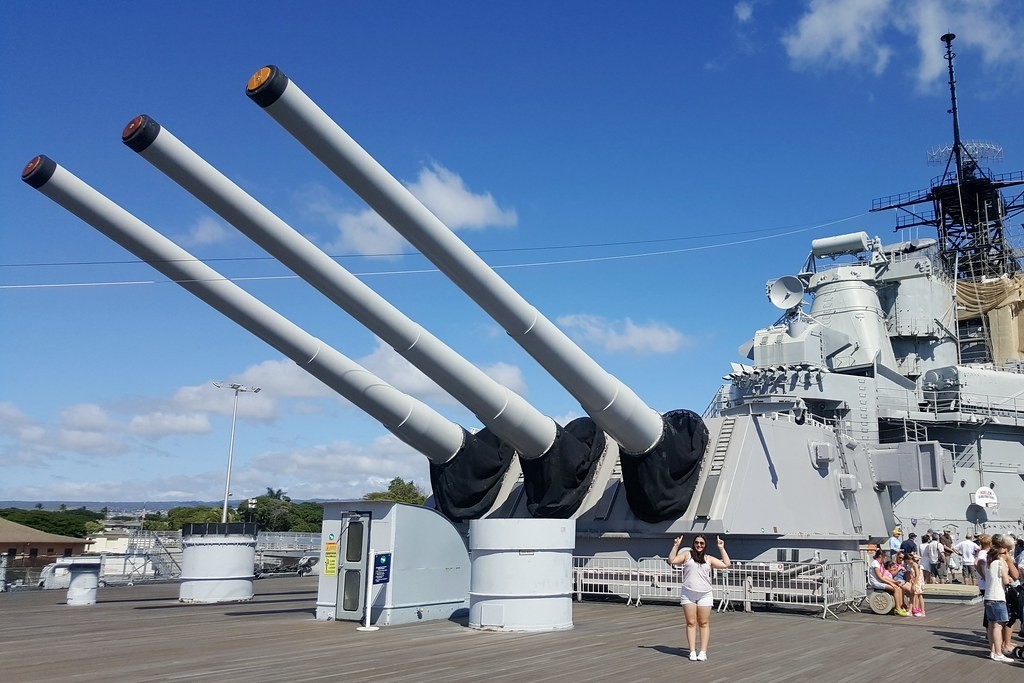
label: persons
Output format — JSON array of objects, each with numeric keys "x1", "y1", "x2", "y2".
[
  {"x1": 668, "y1": 535, "x2": 731, "y2": 661},
  {"x1": 868, "y1": 528, "x2": 1024, "y2": 662}
]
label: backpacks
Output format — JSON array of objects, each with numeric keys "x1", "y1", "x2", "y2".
[{"x1": 1006, "y1": 585, "x2": 1024, "y2": 628}]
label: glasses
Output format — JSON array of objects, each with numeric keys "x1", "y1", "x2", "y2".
[
  {"x1": 999, "y1": 551, "x2": 1006, "y2": 555},
  {"x1": 694, "y1": 541, "x2": 705, "y2": 545},
  {"x1": 899, "y1": 557, "x2": 902, "y2": 559}
]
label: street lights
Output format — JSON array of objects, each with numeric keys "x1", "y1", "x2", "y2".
[{"x1": 212, "y1": 380, "x2": 261, "y2": 522}]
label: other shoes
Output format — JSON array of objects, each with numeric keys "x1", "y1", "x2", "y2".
[
  {"x1": 912, "y1": 608, "x2": 925, "y2": 617},
  {"x1": 903, "y1": 603, "x2": 909, "y2": 610},
  {"x1": 1002, "y1": 646, "x2": 1015, "y2": 652},
  {"x1": 952, "y1": 579, "x2": 962, "y2": 584}
]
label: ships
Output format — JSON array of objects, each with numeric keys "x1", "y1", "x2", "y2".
[{"x1": 22, "y1": 34, "x2": 1024, "y2": 612}]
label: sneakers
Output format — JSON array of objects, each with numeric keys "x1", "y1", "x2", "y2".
[
  {"x1": 689, "y1": 650, "x2": 708, "y2": 661},
  {"x1": 990, "y1": 652, "x2": 1014, "y2": 662}
]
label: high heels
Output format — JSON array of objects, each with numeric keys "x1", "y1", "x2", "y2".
[{"x1": 894, "y1": 607, "x2": 907, "y2": 616}]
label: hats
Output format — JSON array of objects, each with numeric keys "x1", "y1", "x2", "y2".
[
  {"x1": 892, "y1": 530, "x2": 902, "y2": 535},
  {"x1": 909, "y1": 533, "x2": 918, "y2": 538},
  {"x1": 927, "y1": 528, "x2": 933, "y2": 533}
]
